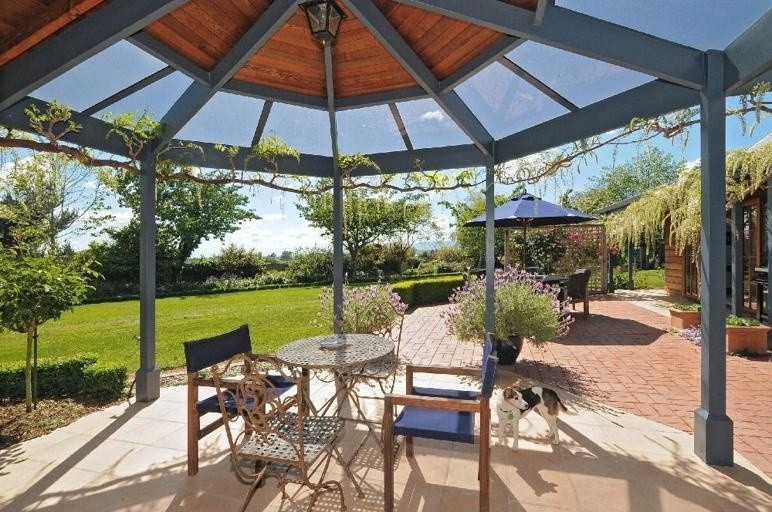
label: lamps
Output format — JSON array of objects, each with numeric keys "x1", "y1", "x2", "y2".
[{"x1": 298, "y1": 0, "x2": 348, "y2": 46}]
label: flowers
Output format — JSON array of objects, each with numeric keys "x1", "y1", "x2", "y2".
[
  {"x1": 309, "y1": 281, "x2": 408, "y2": 333},
  {"x1": 437, "y1": 263, "x2": 576, "y2": 352}
]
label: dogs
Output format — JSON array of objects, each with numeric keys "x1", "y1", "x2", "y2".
[{"x1": 496, "y1": 385, "x2": 580, "y2": 455}]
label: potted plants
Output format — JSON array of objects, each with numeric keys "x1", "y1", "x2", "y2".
[
  {"x1": 669, "y1": 302, "x2": 701, "y2": 329},
  {"x1": 725, "y1": 313, "x2": 772, "y2": 356}
]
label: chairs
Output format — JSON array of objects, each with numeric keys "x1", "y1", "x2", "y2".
[
  {"x1": 563, "y1": 269, "x2": 592, "y2": 319},
  {"x1": 209, "y1": 351, "x2": 363, "y2": 511},
  {"x1": 335, "y1": 300, "x2": 403, "y2": 433},
  {"x1": 525, "y1": 267, "x2": 543, "y2": 276},
  {"x1": 383, "y1": 331, "x2": 499, "y2": 510},
  {"x1": 181, "y1": 323, "x2": 311, "y2": 487}
]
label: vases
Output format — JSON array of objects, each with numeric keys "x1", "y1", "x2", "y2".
[{"x1": 494, "y1": 336, "x2": 525, "y2": 365}]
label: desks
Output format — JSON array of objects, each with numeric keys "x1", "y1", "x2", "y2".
[
  {"x1": 272, "y1": 331, "x2": 396, "y2": 500},
  {"x1": 531, "y1": 276, "x2": 562, "y2": 303}
]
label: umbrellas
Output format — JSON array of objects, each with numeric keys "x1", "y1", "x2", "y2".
[{"x1": 461, "y1": 190, "x2": 597, "y2": 281}]
